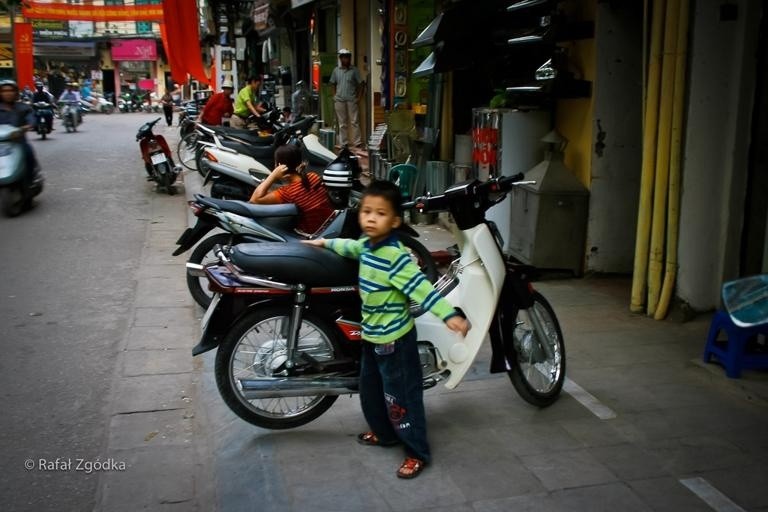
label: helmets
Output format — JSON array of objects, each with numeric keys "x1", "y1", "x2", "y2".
[
  {"x1": 63, "y1": 82, "x2": 79, "y2": 88},
  {"x1": 34, "y1": 80, "x2": 44, "y2": 88},
  {"x1": 0, "y1": 79, "x2": 17, "y2": 88}
]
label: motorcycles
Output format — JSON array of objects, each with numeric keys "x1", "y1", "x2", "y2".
[
  {"x1": 133, "y1": 117, "x2": 181, "y2": 196},
  {"x1": 0, "y1": 125, "x2": 44, "y2": 218},
  {"x1": 18, "y1": 89, "x2": 163, "y2": 140}
]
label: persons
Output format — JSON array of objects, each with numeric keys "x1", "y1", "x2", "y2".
[
  {"x1": 0, "y1": 79, "x2": 44, "y2": 193},
  {"x1": 328, "y1": 50, "x2": 366, "y2": 146},
  {"x1": 301, "y1": 180, "x2": 469, "y2": 478},
  {"x1": 194, "y1": 78, "x2": 233, "y2": 127},
  {"x1": 59, "y1": 75, "x2": 101, "y2": 131},
  {"x1": 282, "y1": 105, "x2": 297, "y2": 127},
  {"x1": 160, "y1": 87, "x2": 174, "y2": 126},
  {"x1": 32, "y1": 80, "x2": 55, "y2": 116},
  {"x1": 249, "y1": 146, "x2": 336, "y2": 238},
  {"x1": 228, "y1": 72, "x2": 267, "y2": 131}
]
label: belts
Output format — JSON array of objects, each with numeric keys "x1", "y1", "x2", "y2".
[{"x1": 232, "y1": 113, "x2": 247, "y2": 120}]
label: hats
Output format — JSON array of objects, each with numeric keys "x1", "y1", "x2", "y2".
[
  {"x1": 220, "y1": 80, "x2": 234, "y2": 91},
  {"x1": 337, "y1": 48, "x2": 351, "y2": 58}
]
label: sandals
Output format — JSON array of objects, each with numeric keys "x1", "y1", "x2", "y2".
[
  {"x1": 396, "y1": 456, "x2": 425, "y2": 479},
  {"x1": 357, "y1": 430, "x2": 380, "y2": 446}
]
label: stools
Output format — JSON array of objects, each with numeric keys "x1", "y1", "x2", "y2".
[{"x1": 702, "y1": 308, "x2": 767, "y2": 381}]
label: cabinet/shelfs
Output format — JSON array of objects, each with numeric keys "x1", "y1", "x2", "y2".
[{"x1": 499, "y1": 1, "x2": 595, "y2": 105}]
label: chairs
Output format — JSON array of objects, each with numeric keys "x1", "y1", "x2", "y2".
[{"x1": 385, "y1": 164, "x2": 418, "y2": 224}]
